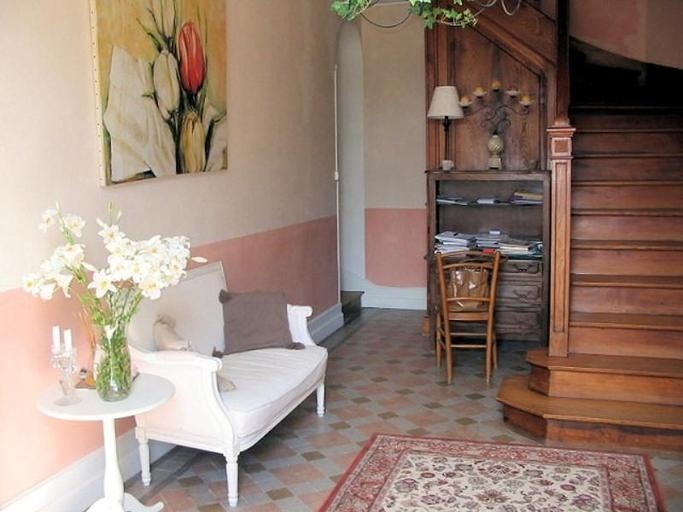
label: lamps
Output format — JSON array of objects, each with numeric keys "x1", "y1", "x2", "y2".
[
  {"x1": 459, "y1": 80, "x2": 534, "y2": 170},
  {"x1": 423, "y1": 86, "x2": 464, "y2": 174}
]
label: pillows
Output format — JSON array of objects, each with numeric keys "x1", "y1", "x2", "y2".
[
  {"x1": 151, "y1": 311, "x2": 236, "y2": 393},
  {"x1": 212, "y1": 288, "x2": 306, "y2": 359}
]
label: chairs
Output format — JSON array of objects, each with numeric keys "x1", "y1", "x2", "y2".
[
  {"x1": 435, "y1": 250, "x2": 501, "y2": 385},
  {"x1": 90, "y1": 259, "x2": 329, "y2": 507}
]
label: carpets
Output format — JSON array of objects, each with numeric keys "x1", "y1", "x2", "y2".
[{"x1": 318, "y1": 432, "x2": 667, "y2": 511}]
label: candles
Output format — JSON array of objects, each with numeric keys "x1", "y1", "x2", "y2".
[
  {"x1": 64, "y1": 328, "x2": 72, "y2": 352},
  {"x1": 52, "y1": 325, "x2": 61, "y2": 350}
]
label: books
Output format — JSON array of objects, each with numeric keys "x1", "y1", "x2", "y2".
[{"x1": 432, "y1": 188, "x2": 542, "y2": 256}]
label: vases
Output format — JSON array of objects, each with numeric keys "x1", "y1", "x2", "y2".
[{"x1": 91, "y1": 324, "x2": 132, "y2": 402}]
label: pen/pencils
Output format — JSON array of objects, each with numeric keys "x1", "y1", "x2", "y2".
[{"x1": 454, "y1": 232, "x2": 459, "y2": 236}]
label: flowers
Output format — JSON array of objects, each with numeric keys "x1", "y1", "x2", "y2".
[{"x1": 22, "y1": 200, "x2": 191, "y2": 397}]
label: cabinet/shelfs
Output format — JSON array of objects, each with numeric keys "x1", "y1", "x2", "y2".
[{"x1": 423, "y1": 171, "x2": 550, "y2": 346}]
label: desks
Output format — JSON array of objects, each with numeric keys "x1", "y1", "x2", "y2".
[{"x1": 38, "y1": 373, "x2": 165, "y2": 512}]
